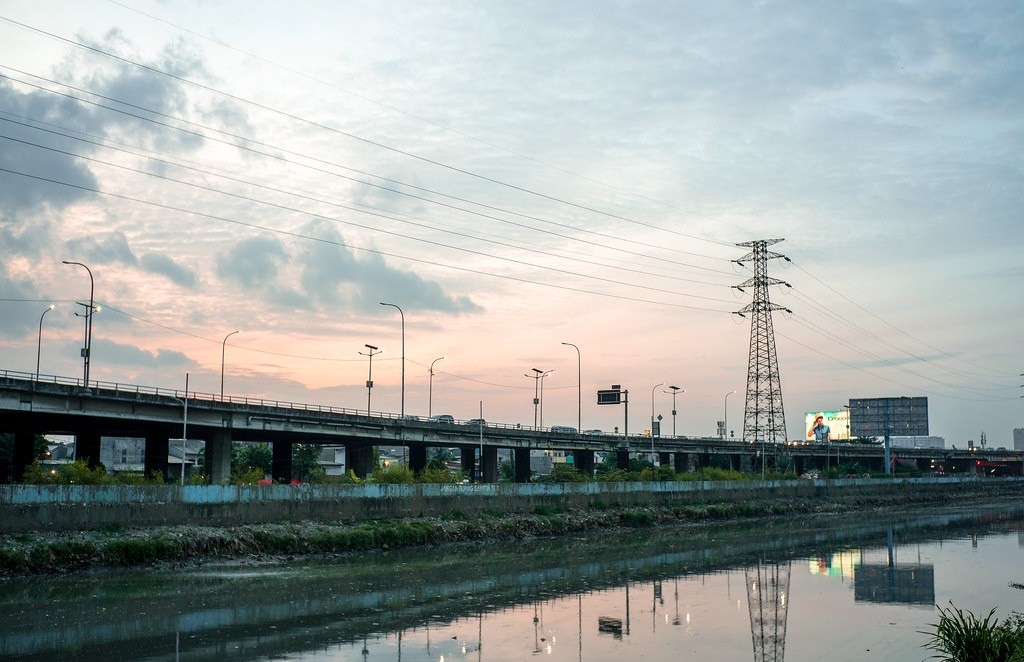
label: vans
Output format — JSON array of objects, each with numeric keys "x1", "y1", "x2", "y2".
[{"x1": 552, "y1": 426, "x2": 576, "y2": 433}]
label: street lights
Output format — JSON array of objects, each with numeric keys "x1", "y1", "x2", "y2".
[
  {"x1": 83, "y1": 307, "x2": 101, "y2": 386},
  {"x1": 562, "y1": 342, "x2": 580, "y2": 434},
  {"x1": 663, "y1": 385, "x2": 685, "y2": 438},
  {"x1": 63, "y1": 261, "x2": 93, "y2": 386},
  {"x1": 524, "y1": 368, "x2": 548, "y2": 431},
  {"x1": 171, "y1": 395, "x2": 187, "y2": 485},
  {"x1": 540, "y1": 370, "x2": 556, "y2": 431},
  {"x1": 37, "y1": 305, "x2": 55, "y2": 381},
  {"x1": 380, "y1": 302, "x2": 404, "y2": 419},
  {"x1": 844, "y1": 405, "x2": 849, "y2": 441},
  {"x1": 358, "y1": 344, "x2": 382, "y2": 416},
  {"x1": 221, "y1": 330, "x2": 240, "y2": 402},
  {"x1": 725, "y1": 391, "x2": 735, "y2": 438},
  {"x1": 652, "y1": 383, "x2": 664, "y2": 422},
  {"x1": 430, "y1": 357, "x2": 444, "y2": 418}
]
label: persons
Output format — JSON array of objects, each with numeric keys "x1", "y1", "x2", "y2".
[{"x1": 808, "y1": 415, "x2": 832, "y2": 440}]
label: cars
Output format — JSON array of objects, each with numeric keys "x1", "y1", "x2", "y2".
[
  {"x1": 997, "y1": 447, "x2": 1006, "y2": 451},
  {"x1": 584, "y1": 430, "x2": 602, "y2": 435},
  {"x1": 399, "y1": 415, "x2": 419, "y2": 421},
  {"x1": 431, "y1": 415, "x2": 454, "y2": 424},
  {"x1": 789, "y1": 440, "x2": 803, "y2": 445},
  {"x1": 465, "y1": 419, "x2": 485, "y2": 426},
  {"x1": 802, "y1": 440, "x2": 815, "y2": 445}
]
